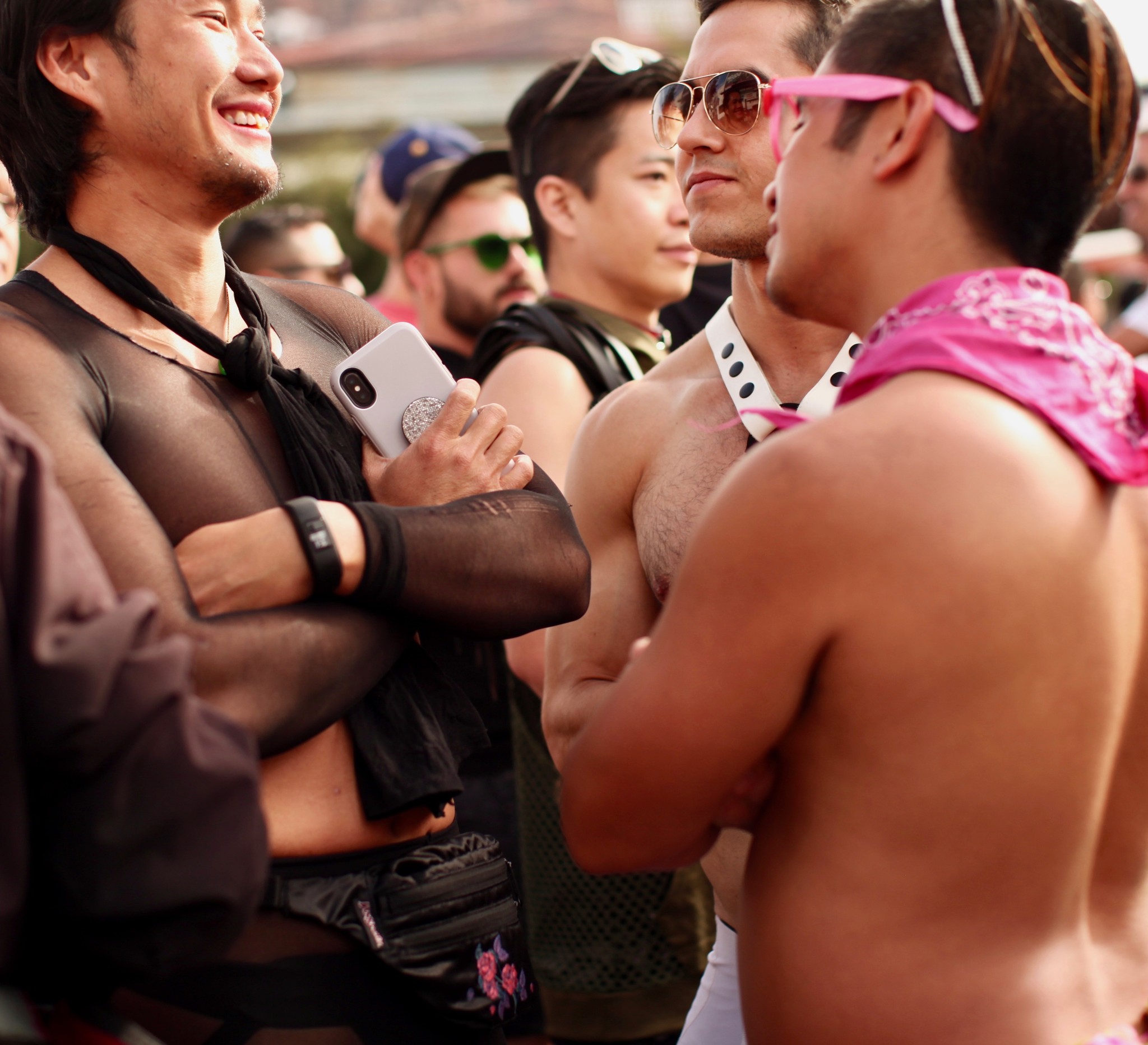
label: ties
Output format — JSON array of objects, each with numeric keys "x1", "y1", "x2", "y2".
[
  {"x1": 46, "y1": 218, "x2": 460, "y2": 822},
  {"x1": 737, "y1": 268, "x2": 1148, "y2": 487}
]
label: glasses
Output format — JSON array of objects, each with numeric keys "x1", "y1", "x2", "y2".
[
  {"x1": 648, "y1": 72, "x2": 774, "y2": 151},
  {"x1": 756, "y1": 73, "x2": 977, "y2": 162},
  {"x1": 264, "y1": 254, "x2": 354, "y2": 290},
  {"x1": 425, "y1": 232, "x2": 544, "y2": 271},
  {"x1": 521, "y1": 37, "x2": 665, "y2": 180},
  {"x1": 1123, "y1": 166, "x2": 1146, "y2": 184}
]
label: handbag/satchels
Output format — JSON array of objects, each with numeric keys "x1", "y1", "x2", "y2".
[{"x1": 353, "y1": 832, "x2": 535, "y2": 1043}]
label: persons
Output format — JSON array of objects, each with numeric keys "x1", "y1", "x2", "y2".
[{"x1": 0, "y1": 1, "x2": 1148, "y2": 1045}]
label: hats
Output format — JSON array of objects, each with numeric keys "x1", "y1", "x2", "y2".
[{"x1": 383, "y1": 123, "x2": 478, "y2": 205}]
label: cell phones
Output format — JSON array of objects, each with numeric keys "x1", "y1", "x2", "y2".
[{"x1": 331, "y1": 322, "x2": 517, "y2": 477}]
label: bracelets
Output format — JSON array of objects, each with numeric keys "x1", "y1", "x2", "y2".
[{"x1": 282, "y1": 494, "x2": 341, "y2": 602}]
